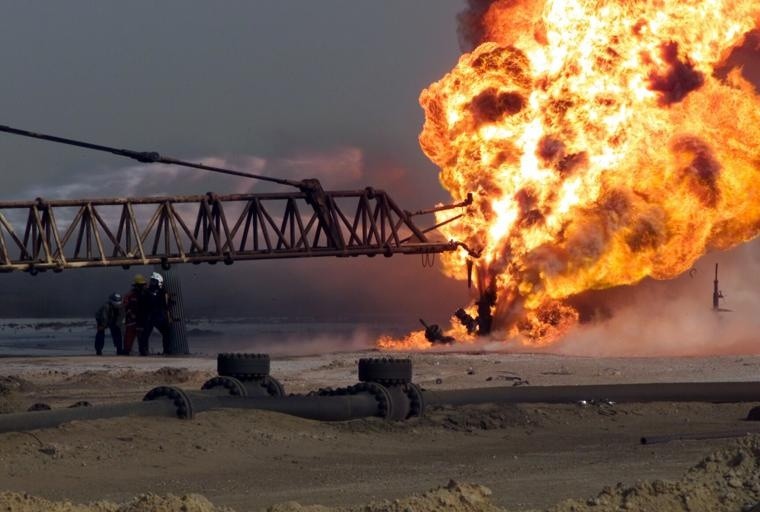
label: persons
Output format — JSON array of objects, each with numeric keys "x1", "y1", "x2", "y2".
[
  {"x1": 138, "y1": 272, "x2": 170, "y2": 357},
  {"x1": 123, "y1": 275, "x2": 148, "y2": 355},
  {"x1": 93, "y1": 292, "x2": 125, "y2": 356}
]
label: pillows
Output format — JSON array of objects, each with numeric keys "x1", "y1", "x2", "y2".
[
  {"x1": 134, "y1": 272, "x2": 163, "y2": 288},
  {"x1": 109, "y1": 293, "x2": 122, "y2": 305}
]
